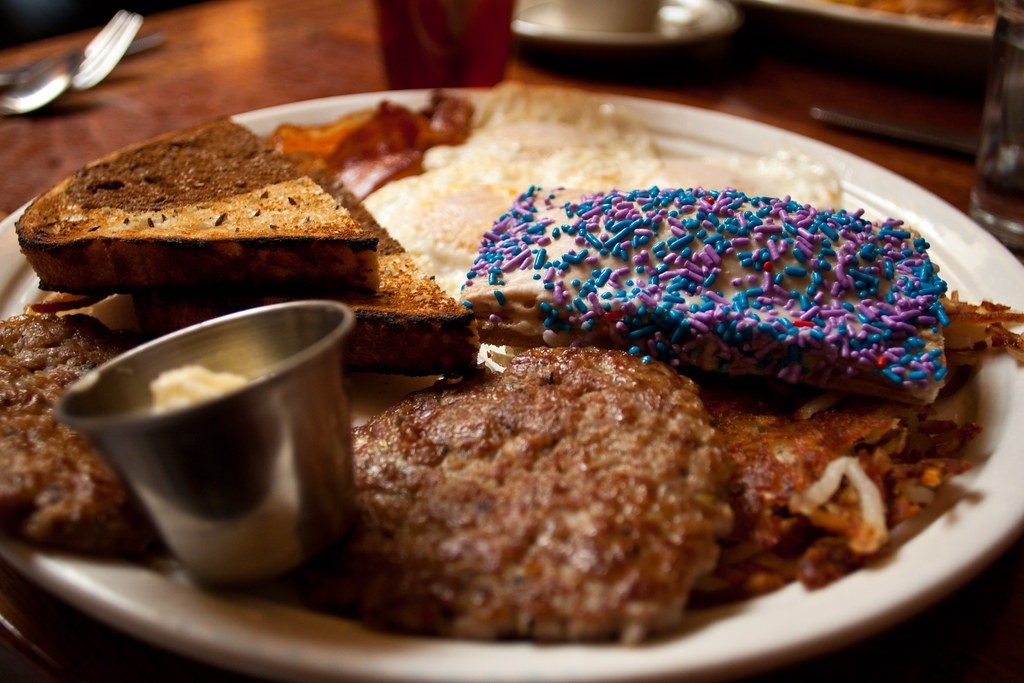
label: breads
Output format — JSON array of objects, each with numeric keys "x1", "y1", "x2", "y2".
[
  {"x1": 465, "y1": 188, "x2": 945, "y2": 399},
  {"x1": 51, "y1": 155, "x2": 480, "y2": 375},
  {"x1": 13, "y1": 121, "x2": 381, "y2": 289}
]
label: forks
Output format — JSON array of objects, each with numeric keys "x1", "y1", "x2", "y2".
[{"x1": 71, "y1": 10, "x2": 144, "y2": 93}]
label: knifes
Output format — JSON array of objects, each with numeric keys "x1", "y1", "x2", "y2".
[
  {"x1": 809, "y1": 106, "x2": 980, "y2": 157},
  {"x1": 0, "y1": 27, "x2": 169, "y2": 88}
]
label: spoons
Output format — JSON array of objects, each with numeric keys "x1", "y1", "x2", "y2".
[{"x1": 0, "y1": 46, "x2": 83, "y2": 115}]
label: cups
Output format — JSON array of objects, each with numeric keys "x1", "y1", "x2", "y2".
[
  {"x1": 54, "y1": 302, "x2": 358, "y2": 598},
  {"x1": 969, "y1": 0, "x2": 1024, "y2": 251},
  {"x1": 558, "y1": 0, "x2": 664, "y2": 32},
  {"x1": 367, "y1": 0, "x2": 517, "y2": 89}
]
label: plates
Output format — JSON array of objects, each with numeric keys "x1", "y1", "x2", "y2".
[
  {"x1": 0, "y1": 86, "x2": 1024, "y2": 683},
  {"x1": 510, "y1": 0, "x2": 745, "y2": 57},
  {"x1": 730, "y1": 0, "x2": 992, "y2": 78}
]
